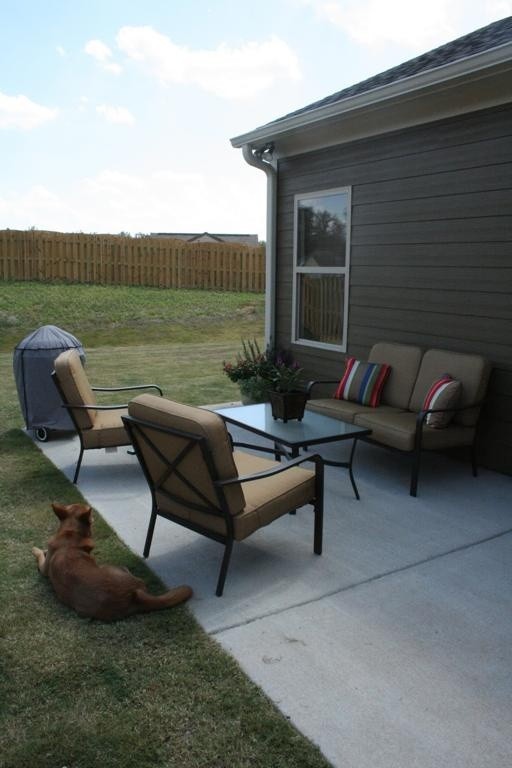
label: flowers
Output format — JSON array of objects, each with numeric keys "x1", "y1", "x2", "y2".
[
  {"x1": 222, "y1": 334, "x2": 295, "y2": 403},
  {"x1": 237, "y1": 353, "x2": 304, "y2": 401}
]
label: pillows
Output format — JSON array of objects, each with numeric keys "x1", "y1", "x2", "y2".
[
  {"x1": 422, "y1": 374, "x2": 463, "y2": 430},
  {"x1": 333, "y1": 355, "x2": 392, "y2": 408}
]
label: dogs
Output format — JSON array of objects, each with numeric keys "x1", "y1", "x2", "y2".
[{"x1": 31, "y1": 503, "x2": 193, "y2": 622}]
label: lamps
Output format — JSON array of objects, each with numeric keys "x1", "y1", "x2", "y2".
[
  {"x1": 253, "y1": 146, "x2": 266, "y2": 162},
  {"x1": 261, "y1": 141, "x2": 275, "y2": 162}
]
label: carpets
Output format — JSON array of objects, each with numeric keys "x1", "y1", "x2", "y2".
[
  {"x1": 21, "y1": 400, "x2": 512, "y2": 634},
  {"x1": 208, "y1": 529, "x2": 512, "y2": 768}
]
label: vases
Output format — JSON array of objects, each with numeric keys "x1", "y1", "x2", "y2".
[
  {"x1": 240, "y1": 387, "x2": 259, "y2": 407},
  {"x1": 267, "y1": 390, "x2": 309, "y2": 424}
]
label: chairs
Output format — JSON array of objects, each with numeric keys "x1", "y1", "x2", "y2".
[
  {"x1": 51, "y1": 348, "x2": 163, "y2": 484},
  {"x1": 120, "y1": 394, "x2": 317, "y2": 598},
  {"x1": 303, "y1": 342, "x2": 492, "y2": 497}
]
